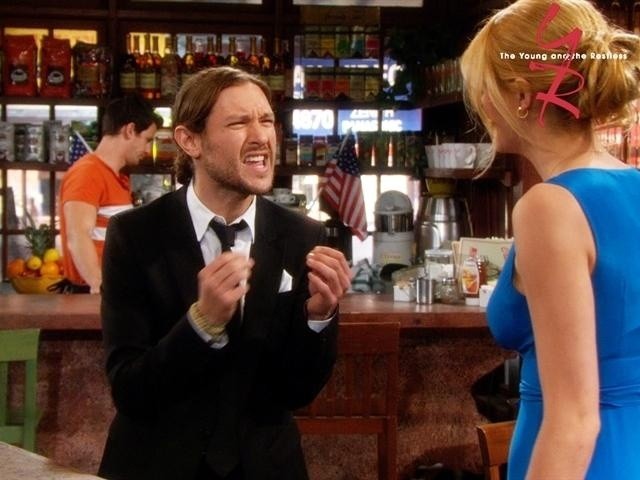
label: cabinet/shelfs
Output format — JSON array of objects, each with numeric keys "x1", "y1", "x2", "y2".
[
  {"x1": 1, "y1": 0, "x2": 283, "y2": 283},
  {"x1": 422, "y1": 1, "x2": 544, "y2": 290},
  {"x1": 284, "y1": 0, "x2": 421, "y2": 267}
]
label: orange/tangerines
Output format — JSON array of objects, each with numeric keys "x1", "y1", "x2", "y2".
[
  {"x1": 40, "y1": 262, "x2": 58, "y2": 276},
  {"x1": 7, "y1": 258, "x2": 26, "y2": 279}
]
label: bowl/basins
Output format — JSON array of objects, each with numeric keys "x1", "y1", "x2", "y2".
[{"x1": 426, "y1": 178, "x2": 451, "y2": 197}]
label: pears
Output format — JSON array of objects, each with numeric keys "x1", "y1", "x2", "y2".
[
  {"x1": 27, "y1": 256, "x2": 41, "y2": 270},
  {"x1": 44, "y1": 248, "x2": 58, "y2": 262}
]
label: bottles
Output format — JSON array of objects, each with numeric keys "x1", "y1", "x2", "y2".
[
  {"x1": 439, "y1": 276, "x2": 460, "y2": 306},
  {"x1": 225, "y1": 38, "x2": 240, "y2": 68},
  {"x1": 137, "y1": 34, "x2": 156, "y2": 102},
  {"x1": 299, "y1": 137, "x2": 315, "y2": 167},
  {"x1": 117, "y1": 33, "x2": 136, "y2": 99},
  {"x1": 267, "y1": 38, "x2": 286, "y2": 109},
  {"x1": 256, "y1": 40, "x2": 270, "y2": 75},
  {"x1": 160, "y1": 38, "x2": 179, "y2": 102},
  {"x1": 313, "y1": 136, "x2": 330, "y2": 167},
  {"x1": 284, "y1": 134, "x2": 298, "y2": 166},
  {"x1": 151, "y1": 36, "x2": 161, "y2": 100},
  {"x1": 215, "y1": 34, "x2": 226, "y2": 66},
  {"x1": 246, "y1": 38, "x2": 261, "y2": 70},
  {"x1": 200, "y1": 37, "x2": 218, "y2": 71},
  {"x1": 179, "y1": 35, "x2": 199, "y2": 86},
  {"x1": 173, "y1": 36, "x2": 181, "y2": 68},
  {"x1": 132, "y1": 34, "x2": 141, "y2": 66}
]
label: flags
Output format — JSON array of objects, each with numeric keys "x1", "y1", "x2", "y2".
[
  {"x1": 318, "y1": 131, "x2": 370, "y2": 242},
  {"x1": 68, "y1": 129, "x2": 94, "y2": 163}
]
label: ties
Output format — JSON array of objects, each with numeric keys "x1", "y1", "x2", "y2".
[{"x1": 209, "y1": 220, "x2": 246, "y2": 336}]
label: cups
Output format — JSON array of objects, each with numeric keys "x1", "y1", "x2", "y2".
[
  {"x1": 425, "y1": 142, "x2": 477, "y2": 169},
  {"x1": 278, "y1": 194, "x2": 295, "y2": 203},
  {"x1": 273, "y1": 188, "x2": 292, "y2": 197}
]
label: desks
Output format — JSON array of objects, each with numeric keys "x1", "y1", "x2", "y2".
[{"x1": 2, "y1": 294, "x2": 519, "y2": 480}]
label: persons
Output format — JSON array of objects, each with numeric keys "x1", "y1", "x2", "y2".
[
  {"x1": 458, "y1": 1, "x2": 640, "y2": 480},
  {"x1": 98, "y1": 66, "x2": 353, "y2": 480},
  {"x1": 56, "y1": 95, "x2": 164, "y2": 294}
]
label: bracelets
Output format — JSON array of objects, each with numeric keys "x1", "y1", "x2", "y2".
[{"x1": 191, "y1": 302, "x2": 226, "y2": 335}]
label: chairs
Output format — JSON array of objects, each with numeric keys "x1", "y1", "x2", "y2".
[
  {"x1": 477, "y1": 420, "x2": 516, "y2": 480},
  {"x1": 2, "y1": 328, "x2": 45, "y2": 454},
  {"x1": 295, "y1": 321, "x2": 401, "y2": 480}
]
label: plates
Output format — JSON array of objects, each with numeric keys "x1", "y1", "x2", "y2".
[{"x1": 10, "y1": 276, "x2": 66, "y2": 295}]
